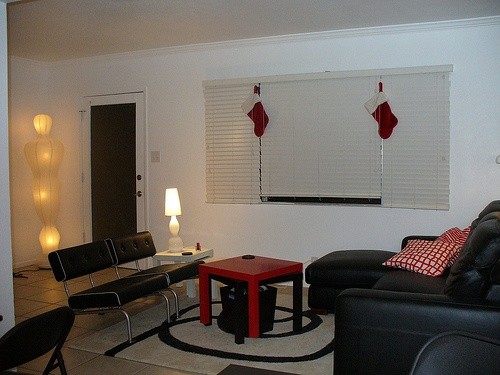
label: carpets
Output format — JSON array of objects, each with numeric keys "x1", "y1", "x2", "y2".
[{"x1": 64, "y1": 296, "x2": 336, "y2": 375}]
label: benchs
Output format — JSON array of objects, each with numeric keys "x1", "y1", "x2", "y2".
[{"x1": 48, "y1": 231, "x2": 206, "y2": 343}]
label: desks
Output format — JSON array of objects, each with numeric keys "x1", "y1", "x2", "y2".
[
  {"x1": 199, "y1": 254, "x2": 303, "y2": 344},
  {"x1": 154, "y1": 248, "x2": 213, "y2": 298}
]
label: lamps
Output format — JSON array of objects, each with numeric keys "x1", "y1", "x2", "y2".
[
  {"x1": 164, "y1": 187, "x2": 183, "y2": 252},
  {"x1": 25, "y1": 113, "x2": 63, "y2": 269}
]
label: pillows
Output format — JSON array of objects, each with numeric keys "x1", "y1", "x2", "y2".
[
  {"x1": 436, "y1": 226, "x2": 471, "y2": 268},
  {"x1": 382, "y1": 239, "x2": 458, "y2": 276}
]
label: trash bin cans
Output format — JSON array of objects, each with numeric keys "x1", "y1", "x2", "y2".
[{"x1": 220, "y1": 285, "x2": 277, "y2": 334}]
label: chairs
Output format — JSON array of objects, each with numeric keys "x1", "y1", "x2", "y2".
[{"x1": 0, "y1": 305, "x2": 75, "y2": 375}]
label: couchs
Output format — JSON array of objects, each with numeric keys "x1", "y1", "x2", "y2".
[{"x1": 304, "y1": 198, "x2": 500, "y2": 375}]
